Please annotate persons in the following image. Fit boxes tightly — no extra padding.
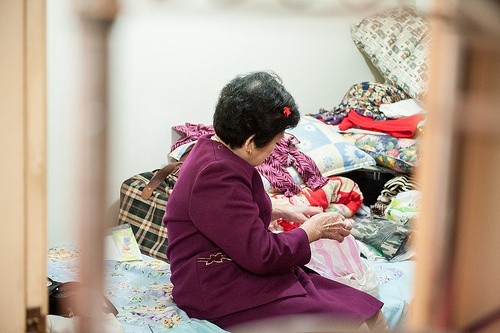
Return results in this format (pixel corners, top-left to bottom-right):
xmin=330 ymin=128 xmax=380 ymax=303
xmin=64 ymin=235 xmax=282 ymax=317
xmin=162 ymin=71 xmax=391 ymax=332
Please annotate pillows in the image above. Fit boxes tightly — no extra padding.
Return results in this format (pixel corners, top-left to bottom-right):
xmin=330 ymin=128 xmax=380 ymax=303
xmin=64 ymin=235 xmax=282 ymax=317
xmin=351 ymin=6 xmax=428 ymax=108
xmin=170 ymin=115 xmax=376 ymax=198
xmin=356 ymin=134 xmax=417 ymax=175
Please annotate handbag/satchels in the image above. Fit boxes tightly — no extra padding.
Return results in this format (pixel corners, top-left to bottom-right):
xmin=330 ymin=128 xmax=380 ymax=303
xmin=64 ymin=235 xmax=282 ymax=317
xmin=118 ymin=160 xmax=186 ymax=262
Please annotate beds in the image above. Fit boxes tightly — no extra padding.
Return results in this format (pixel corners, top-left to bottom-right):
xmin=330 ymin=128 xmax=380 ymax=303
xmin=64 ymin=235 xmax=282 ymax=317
xmin=45 ymin=6 xmax=428 ymax=333
